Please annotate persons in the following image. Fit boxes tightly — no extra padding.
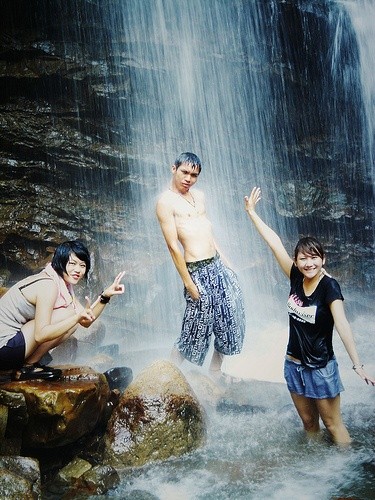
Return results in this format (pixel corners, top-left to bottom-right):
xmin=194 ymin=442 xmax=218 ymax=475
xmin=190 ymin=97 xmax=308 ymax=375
xmin=155 ymin=152 xmax=244 ymax=379
xmin=244 ymin=185 xmax=374 ymax=445
xmin=0 ymin=240 xmax=128 ymax=385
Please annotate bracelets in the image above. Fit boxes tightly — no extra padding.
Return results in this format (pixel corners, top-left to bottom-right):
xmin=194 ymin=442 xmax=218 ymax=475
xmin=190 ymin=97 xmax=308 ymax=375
xmin=96 ymin=291 xmax=110 ymax=305
xmin=350 ymin=362 xmax=364 ymax=369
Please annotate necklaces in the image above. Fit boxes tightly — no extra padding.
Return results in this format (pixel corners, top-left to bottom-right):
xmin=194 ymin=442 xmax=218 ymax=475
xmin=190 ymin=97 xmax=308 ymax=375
xmin=170 ymin=184 xmax=196 ymax=208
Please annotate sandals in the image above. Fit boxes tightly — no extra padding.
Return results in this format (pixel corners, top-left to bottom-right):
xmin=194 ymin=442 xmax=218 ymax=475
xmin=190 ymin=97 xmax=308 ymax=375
xmin=8 ymin=363 xmax=63 ymax=381
